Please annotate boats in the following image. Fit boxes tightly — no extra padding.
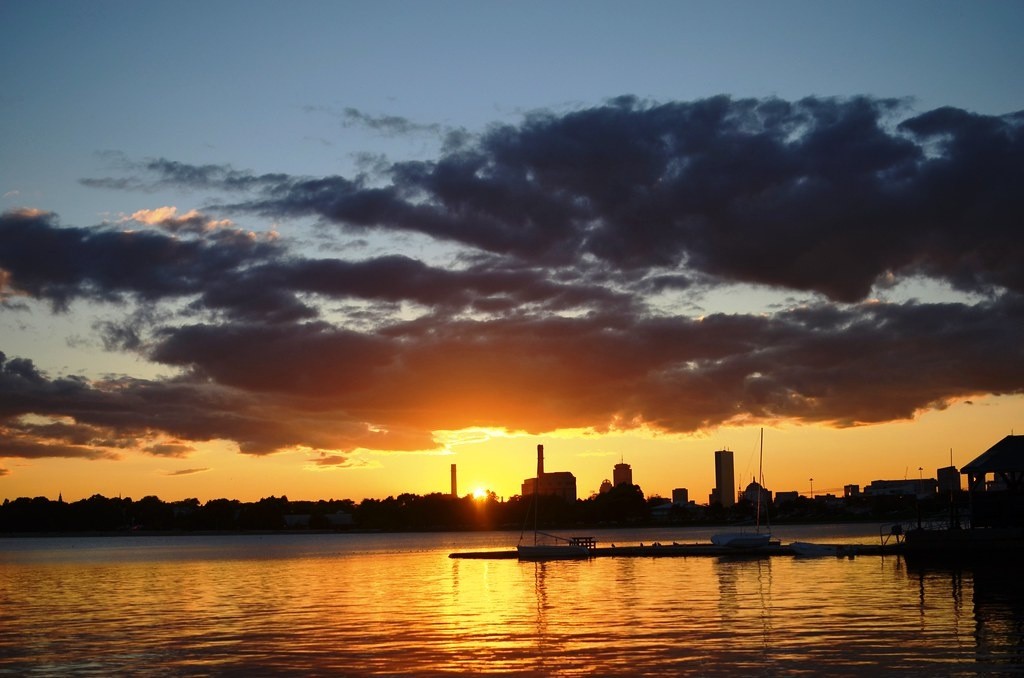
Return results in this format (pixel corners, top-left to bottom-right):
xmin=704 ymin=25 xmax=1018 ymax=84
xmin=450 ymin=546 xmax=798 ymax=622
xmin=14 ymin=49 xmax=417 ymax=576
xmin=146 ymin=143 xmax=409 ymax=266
xmin=904 ymin=525 xmax=1024 ymax=571
xmin=787 ymin=542 xmax=862 ymax=559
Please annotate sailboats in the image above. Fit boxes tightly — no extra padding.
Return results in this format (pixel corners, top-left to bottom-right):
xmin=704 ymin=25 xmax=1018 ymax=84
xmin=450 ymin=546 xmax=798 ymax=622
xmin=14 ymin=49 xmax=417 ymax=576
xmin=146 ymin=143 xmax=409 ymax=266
xmin=517 ymin=478 xmax=591 ymax=560
xmin=710 ymin=429 xmax=776 ymax=549
